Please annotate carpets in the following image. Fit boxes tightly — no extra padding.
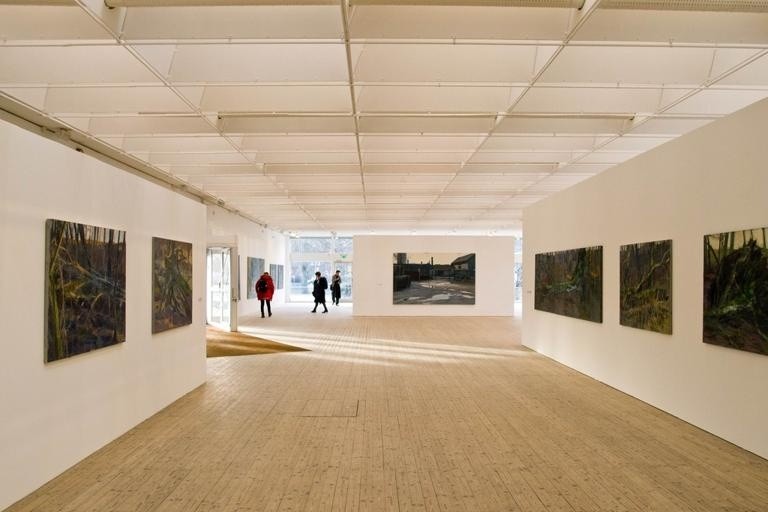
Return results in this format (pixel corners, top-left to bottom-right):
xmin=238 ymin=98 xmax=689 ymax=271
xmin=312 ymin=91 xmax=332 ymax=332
xmin=204 ymin=324 xmax=314 ymax=359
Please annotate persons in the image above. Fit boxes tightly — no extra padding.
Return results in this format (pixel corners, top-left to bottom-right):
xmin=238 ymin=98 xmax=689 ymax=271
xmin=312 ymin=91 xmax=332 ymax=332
xmin=332 ymin=270 xmax=341 ymax=305
xmin=311 ymin=271 xmax=328 ymax=313
xmin=256 ymin=272 xmax=274 ymax=318
